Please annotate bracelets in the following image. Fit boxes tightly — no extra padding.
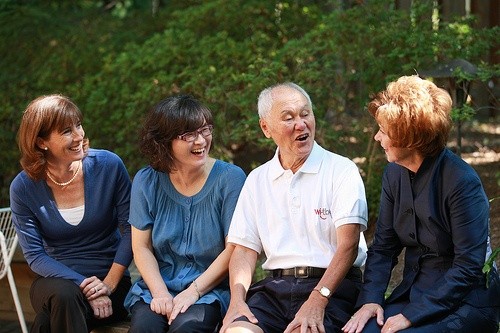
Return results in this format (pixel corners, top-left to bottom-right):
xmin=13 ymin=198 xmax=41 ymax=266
xmin=189 ymin=280 xmax=204 ymax=300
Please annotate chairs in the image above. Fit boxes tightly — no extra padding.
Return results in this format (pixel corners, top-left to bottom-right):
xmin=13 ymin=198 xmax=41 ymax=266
xmin=0 ymin=208 xmax=30 ymax=333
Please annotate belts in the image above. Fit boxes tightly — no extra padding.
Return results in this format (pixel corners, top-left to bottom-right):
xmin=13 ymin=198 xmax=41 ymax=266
xmin=269 ymin=267 xmax=350 ymax=279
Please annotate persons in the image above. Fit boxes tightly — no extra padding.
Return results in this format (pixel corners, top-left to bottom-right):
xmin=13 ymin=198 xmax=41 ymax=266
xmin=127 ymin=96 xmax=247 ymax=333
xmin=340 ymin=74 xmax=499 ymax=333
xmin=11 ymin=93 xmax=135 ymax=333
xmin=218 ymin=80 xmax=368 ymax=332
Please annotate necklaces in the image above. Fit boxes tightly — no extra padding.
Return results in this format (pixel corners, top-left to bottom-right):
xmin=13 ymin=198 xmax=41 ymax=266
xmin=45 ymin=160 xmax=83 ymax=187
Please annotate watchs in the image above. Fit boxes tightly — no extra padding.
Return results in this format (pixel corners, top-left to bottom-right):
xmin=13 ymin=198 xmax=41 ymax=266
xmin=314 ymin=284 xmax=333 ymax=300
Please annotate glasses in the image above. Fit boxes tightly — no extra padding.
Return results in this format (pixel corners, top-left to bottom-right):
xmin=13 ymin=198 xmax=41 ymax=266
xmin=176 ymin=123 xmax=213 ymax=142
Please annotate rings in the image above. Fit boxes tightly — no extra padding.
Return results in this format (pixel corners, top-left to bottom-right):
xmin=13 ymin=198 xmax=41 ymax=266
xmin=93 ymin=286 xmax=98 ymax=293
xmin=388 ymin=326 xmax=393 ymax=331
xmin=350 ymin=315 xmax=356 ymax=320
